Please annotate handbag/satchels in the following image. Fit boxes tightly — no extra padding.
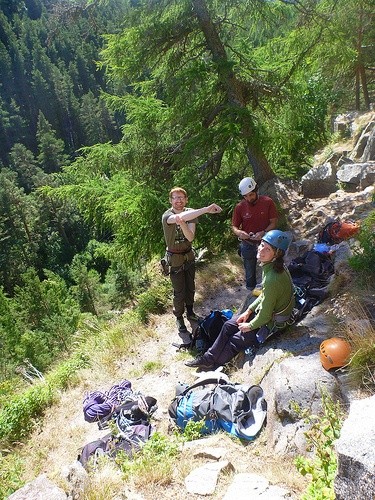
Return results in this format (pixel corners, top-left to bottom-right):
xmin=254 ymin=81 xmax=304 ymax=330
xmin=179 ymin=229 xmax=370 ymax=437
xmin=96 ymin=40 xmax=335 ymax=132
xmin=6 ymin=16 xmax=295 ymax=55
xmin=79 ymin=378 xmax=159 ymax=472
xmin=317 ymin=221 xmax=359 ymax=245
xmin=192 ymin=310 xmax=233 ymax=353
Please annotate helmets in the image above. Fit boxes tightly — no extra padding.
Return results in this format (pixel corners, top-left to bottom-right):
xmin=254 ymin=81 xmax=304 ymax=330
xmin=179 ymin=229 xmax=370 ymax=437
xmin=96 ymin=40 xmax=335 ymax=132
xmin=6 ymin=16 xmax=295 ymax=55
xmin=239 ymin=177 xmax=257 ymax=195
xmin=262 ymin=230 xmax=290 ymax=252
xmin=320 ymin=338 xmax=351 ymax=371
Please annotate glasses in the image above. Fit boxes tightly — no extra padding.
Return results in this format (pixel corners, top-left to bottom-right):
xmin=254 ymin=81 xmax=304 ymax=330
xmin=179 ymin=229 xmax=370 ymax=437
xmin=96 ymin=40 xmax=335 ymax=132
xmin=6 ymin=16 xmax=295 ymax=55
xmin=171 ymin=196 xmax=186 ymax=200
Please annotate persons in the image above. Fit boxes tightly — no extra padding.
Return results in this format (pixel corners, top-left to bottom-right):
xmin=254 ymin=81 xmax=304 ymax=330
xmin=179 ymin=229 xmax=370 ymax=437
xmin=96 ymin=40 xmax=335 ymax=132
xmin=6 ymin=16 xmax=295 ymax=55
xmin=162 ymin=188 xmax=223 ymax=331
xmin=183 ymin=229 xmax=296 ymax=372
xmin=231 ymin=177 xmax=278 ymax=293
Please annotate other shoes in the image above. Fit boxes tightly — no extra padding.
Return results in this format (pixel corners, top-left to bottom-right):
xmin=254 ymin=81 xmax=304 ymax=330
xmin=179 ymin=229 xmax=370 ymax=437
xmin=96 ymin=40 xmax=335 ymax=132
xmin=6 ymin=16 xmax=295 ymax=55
xmin=176 ymin=318 xmax=187 ymax=332
xmin=186 ymin=312 xmax=203 ymax=321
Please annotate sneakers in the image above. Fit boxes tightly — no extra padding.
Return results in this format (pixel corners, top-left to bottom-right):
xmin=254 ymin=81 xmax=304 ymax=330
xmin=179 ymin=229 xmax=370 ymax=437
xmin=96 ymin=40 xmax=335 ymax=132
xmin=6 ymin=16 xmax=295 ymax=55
xmin=184 ymin=354 xmax=213 ymax=368
xmin=200 ymin=364 xmax=223 ymax=370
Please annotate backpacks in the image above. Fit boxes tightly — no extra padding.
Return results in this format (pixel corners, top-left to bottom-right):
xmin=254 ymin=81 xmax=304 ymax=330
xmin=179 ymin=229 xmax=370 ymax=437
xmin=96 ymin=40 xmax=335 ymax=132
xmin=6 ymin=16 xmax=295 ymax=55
xmin=168 ymin=372 xmax=268 ymax=441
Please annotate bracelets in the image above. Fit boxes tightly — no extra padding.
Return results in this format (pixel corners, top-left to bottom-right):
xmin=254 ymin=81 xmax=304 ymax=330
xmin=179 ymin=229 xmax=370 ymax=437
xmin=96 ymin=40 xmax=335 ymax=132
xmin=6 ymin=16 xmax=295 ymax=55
xmin=248 ymin=323 xmax=252 ymax=331
xmin=264 ymin=230 xmax=266 ymax=232
